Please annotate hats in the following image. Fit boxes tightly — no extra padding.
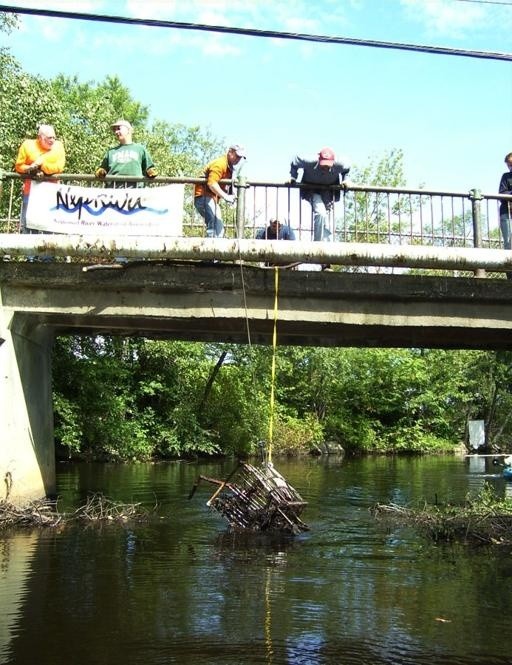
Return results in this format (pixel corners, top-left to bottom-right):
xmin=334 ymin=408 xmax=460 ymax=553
xmin=109 ymin=119 xmax=133 ymax=128
xmin=319 ymin=146 xmax=335 ymax=166
xmin=229 ymin=142 xmax=248 ymax=160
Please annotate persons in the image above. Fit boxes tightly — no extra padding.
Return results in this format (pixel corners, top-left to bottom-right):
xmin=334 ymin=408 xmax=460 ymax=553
xmin=285 ymin=150 xmax=350 ymax=240
xmin=15 ymin=125 xmax=66 ymax=234
xmin=256 ymin=216 xmax=295 ymax=240
xmin=94 ymin=119 xmax=158 ymax=189
xmin=194 ymin=145 xmax=247 ymax=238
xmin=498 ymin=152 xmax=512 ymax=250
